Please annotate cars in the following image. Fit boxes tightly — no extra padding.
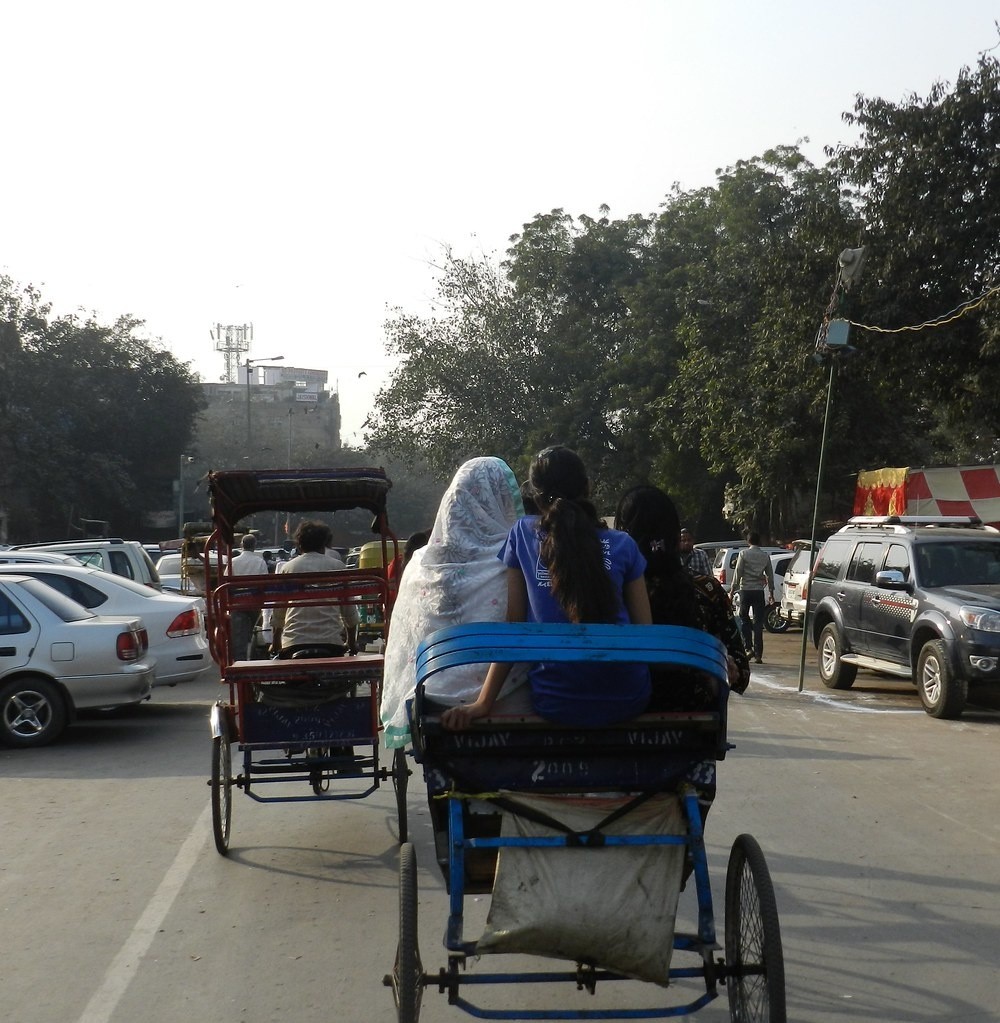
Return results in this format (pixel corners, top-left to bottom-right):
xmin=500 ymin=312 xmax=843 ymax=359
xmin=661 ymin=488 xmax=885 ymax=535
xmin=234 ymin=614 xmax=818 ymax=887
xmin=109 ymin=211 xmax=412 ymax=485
xmin=1 ymin=537 xmax=230 ymax=632
xmin=692 ymin=537 xmax=826 ymax=632
xmin=0 ymin=563 xmax=214 ymax=693
xmin=0 ymin=573 xmax=161 ymax=748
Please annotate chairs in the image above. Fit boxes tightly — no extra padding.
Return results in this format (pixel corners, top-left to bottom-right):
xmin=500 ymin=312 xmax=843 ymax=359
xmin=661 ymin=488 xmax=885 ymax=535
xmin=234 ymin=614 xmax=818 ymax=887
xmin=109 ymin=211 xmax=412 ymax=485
xmin=929 ymin=548 xmax=958 ymax=579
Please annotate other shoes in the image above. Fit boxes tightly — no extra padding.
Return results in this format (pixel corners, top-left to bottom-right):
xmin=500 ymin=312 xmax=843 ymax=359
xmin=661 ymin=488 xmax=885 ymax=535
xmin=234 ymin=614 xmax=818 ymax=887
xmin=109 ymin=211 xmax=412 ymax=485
xmin=755 ymin=657 xmax=762 ymax=663
xmin=283 ymin=746 xmax=304 ymax=755
xmin=339 ymin=764 xmax=363 ymax=778
xmin=746 ymin=649 xmax=754 ymax=660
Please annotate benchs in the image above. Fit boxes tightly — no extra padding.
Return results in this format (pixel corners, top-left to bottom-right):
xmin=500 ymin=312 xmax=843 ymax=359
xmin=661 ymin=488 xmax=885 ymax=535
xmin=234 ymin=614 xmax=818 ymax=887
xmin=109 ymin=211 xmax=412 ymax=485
xmin=417 ymin=703 xmax=723 ymax=826
xmin=226 ymin=654 xmax=385 ymax=744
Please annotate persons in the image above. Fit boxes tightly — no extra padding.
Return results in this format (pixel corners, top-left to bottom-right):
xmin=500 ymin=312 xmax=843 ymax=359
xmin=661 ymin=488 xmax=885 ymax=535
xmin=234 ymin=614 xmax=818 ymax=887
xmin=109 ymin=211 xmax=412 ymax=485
xmin=263 ymin=549 xmax=286 ymax=572
xmin=325 ymin=534 xmax=341 ymax=560
xmin=439 ymin=447 xmax=653 ymax=732
xmin=268 ymin=520 xmax=363 ymax=775
xmin=223 ymin=534 xmax=268 ymax=659
xmin=380 ymin=457 xmax=527 ymax=751
xmin=614 ymin=485 xmax=750 ymax=710
xmin=730 ymin=535 xmax=775 ymax=663
xmin=679 ymin=529 xmax=714 ymax=578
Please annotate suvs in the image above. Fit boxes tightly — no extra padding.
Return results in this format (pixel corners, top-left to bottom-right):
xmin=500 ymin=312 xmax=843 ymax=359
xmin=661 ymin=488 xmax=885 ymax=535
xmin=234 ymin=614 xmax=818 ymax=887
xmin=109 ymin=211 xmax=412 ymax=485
xmin=803 ymin=515 xmax=1000 ymax=719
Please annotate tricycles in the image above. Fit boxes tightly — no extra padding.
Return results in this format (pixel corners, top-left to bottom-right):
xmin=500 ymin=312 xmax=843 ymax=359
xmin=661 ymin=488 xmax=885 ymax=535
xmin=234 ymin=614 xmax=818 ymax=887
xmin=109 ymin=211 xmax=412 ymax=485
xmin=381 ymin=623 xmax=787 ymax=1022
xmin=182 ymin=462 xmax=415 ymax=854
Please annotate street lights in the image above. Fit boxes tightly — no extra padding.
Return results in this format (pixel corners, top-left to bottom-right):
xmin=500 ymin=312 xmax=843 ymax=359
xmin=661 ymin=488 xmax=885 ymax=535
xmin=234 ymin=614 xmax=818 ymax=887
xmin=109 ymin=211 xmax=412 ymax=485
xmin=246 ymin=356 xmax=286 ymax=460
